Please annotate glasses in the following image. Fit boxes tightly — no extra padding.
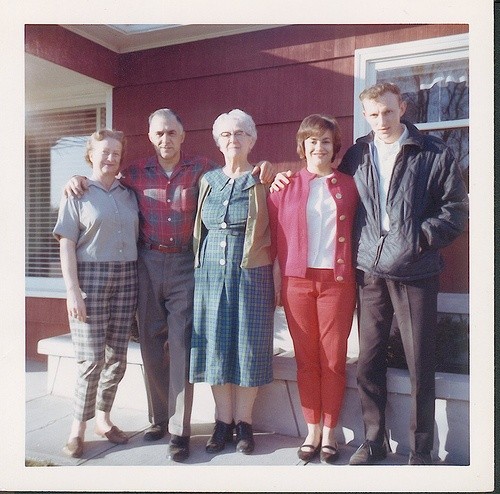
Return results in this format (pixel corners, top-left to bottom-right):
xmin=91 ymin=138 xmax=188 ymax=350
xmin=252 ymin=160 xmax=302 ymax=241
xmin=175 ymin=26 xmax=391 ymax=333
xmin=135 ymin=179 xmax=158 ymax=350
xmin=221 ymin=131 xmax=252 ymax=138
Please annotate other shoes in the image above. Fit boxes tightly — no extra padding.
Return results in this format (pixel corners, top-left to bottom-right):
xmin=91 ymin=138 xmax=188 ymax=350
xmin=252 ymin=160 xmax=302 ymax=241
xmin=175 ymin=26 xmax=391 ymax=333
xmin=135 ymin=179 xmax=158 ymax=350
xmin=144 ymin=424 xmax=167 ymax=440
xmin=320 ymin=441 xmax=338 ymax=461
xmin=409 ymin=450 xmax=432 ymax=465
xmin=94 ymin=425 xmax=128 ymax=444
xmin=63 ymin=437 xmax=85 ymax=459
xmin=349 ymin=439 xmax=387 ymax=465
xmin=169 ymin=434 xmax=190 ymax=462
xmin=206 ymin=417 xmax=235 ymax=451
xmin=235 ymin=419 xmax=254 ymax=453
xmin=297 ymin=432 xmax=321 ymax=459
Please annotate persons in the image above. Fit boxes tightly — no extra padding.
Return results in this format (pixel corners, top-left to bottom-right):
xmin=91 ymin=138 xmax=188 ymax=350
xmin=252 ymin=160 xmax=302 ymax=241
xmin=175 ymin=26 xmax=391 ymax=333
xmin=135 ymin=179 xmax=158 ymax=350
xmin=51 ymin=129 xmax=131 ymax=459
xmin=263 ymin=111 xmax=367 ymax=463
xmin=182 ymin=112 xmax=281 ymax=455
xmin=266 ymin=84 xmax=469 ymax=465
xmin=65 ymin=108 xmax=278 ymax=463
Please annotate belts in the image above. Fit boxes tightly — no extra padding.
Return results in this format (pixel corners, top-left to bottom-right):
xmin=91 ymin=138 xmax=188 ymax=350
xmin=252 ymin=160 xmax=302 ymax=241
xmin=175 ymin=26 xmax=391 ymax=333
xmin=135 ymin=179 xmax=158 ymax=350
xmin=138 ymin=242 xmax=193 ymax=253
